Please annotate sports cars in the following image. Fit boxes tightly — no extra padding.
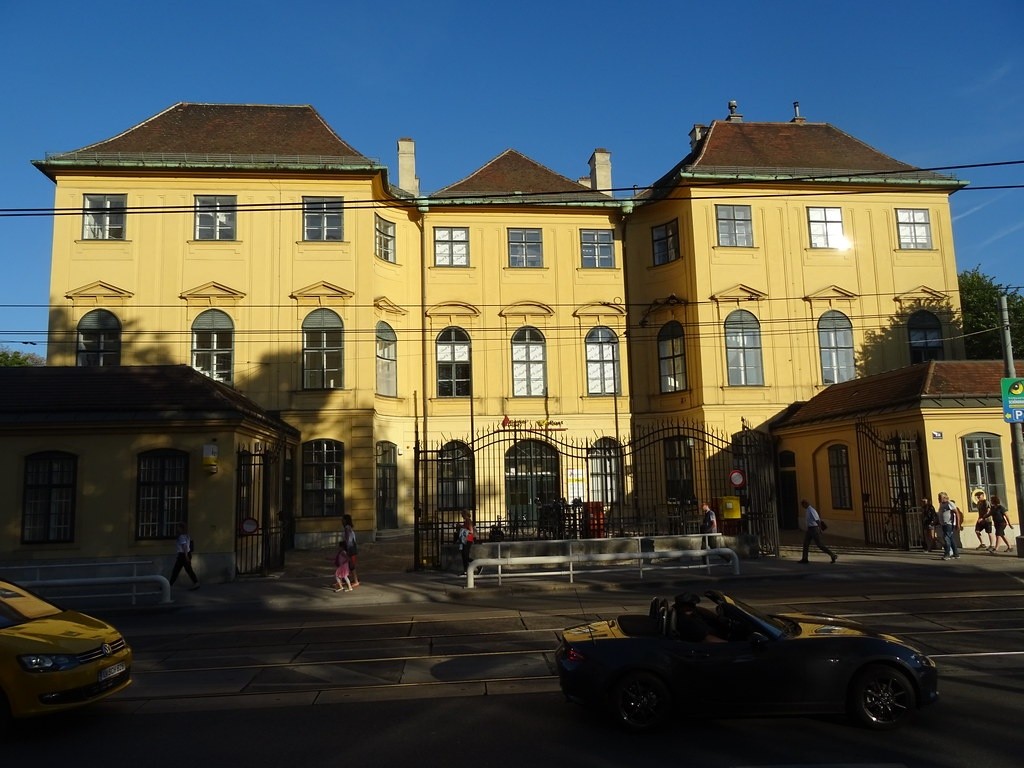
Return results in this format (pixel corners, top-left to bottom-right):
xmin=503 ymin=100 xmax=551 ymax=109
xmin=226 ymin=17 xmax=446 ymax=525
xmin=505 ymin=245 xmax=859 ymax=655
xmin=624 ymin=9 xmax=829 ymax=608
xmin=555 ymin=588 xmax=938 ymax=732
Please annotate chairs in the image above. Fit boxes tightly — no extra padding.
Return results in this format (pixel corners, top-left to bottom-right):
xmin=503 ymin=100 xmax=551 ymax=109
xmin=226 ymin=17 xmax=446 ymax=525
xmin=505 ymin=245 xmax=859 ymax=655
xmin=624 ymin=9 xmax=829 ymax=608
xmin=623 ymin=597 xmax=681 ymax=638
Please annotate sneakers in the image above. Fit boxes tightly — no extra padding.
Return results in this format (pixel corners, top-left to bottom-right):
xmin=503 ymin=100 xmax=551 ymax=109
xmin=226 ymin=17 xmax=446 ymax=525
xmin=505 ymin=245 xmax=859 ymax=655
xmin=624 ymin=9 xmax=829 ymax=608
xmin=830 ymin=555 xmax=837 ymax=563
xmin=989 ymin=548 xmax=998 ymax=554
xmin=986 ymin=546 xmax=994 ymax=550
xmin=976 ymin=544 xmax=987 ymax=550
xmin=477 ymin=566 xmax=483 ymax=574
xmin=950 ymin=555 xmax=960 ymax=559
xmin=941 ymin=556 xmax=951 ymax=560
xmin=1003 ymin=548 xmax=1014 ymax=552
xmin=797 ymin=559 xmax=809 ymax=564
xmin=457 ymin=573 xmax=467 ymax=578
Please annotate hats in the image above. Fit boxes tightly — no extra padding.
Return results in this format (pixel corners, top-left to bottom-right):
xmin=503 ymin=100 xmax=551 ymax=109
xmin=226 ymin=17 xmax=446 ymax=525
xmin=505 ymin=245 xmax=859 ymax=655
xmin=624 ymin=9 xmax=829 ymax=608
xmin=674 ymin=592 xmax=701 ymax=607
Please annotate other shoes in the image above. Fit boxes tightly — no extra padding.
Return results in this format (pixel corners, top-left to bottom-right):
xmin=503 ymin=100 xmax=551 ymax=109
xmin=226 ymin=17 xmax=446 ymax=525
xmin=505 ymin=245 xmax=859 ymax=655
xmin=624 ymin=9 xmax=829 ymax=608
xmin=189 ymin=584 xmax=200 ymax=591
xmin=345 ymin=588 xmax=353 ymax=592
xmin=352 ymin=583 xmax=360 ymax=588
xmin=335 ymin=587 xmax=343 ymax=593
xmin=331 ymin=583 xmax=345 ymax=587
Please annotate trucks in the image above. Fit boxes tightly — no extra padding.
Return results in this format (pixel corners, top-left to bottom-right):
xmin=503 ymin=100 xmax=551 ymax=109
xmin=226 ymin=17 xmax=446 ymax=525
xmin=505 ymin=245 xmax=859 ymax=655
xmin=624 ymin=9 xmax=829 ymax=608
xmin=667 ymin=498 xmax=691 ymax=506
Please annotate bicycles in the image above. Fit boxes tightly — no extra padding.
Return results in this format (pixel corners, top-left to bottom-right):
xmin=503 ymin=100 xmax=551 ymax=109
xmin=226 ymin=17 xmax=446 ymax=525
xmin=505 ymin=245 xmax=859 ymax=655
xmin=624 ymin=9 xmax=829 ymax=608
xmin=500 ymin=510 xmax=537 ymax=536
xmin=563 ymin=507 xmax=574 ymax=528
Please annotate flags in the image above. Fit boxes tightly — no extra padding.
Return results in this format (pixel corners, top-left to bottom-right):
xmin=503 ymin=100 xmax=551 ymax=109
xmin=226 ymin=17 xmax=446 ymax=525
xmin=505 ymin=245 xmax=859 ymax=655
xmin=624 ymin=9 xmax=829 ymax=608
xmin=502 ymin=415 xmax=510 ymax=428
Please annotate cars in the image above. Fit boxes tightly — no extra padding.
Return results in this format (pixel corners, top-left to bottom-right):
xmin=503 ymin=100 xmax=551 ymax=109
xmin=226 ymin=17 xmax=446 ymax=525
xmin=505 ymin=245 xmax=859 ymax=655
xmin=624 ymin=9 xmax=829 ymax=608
xmin=0 ymin=583 xmax=132 ymax=729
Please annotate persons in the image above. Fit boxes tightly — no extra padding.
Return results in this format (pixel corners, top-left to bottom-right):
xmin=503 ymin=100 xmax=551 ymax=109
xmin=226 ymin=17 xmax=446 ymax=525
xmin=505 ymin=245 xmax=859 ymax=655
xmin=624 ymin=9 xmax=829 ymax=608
xmin=938 ymin=491 xmax=960 ymax=561
xmin=532 ymin=498 xmax=568 ymax=539
xmin=169 ymin=522 xmax=200 ymax=590
xmin=701 ymin=501 xmax=733 ymax=572
xmin=675 ymin=592 xmax=727 ymax=644
xmin=333 ymin=515 xmax=359 ymax=592
xmin=975 ymin=492 xmax=1014 ymax=554
xmin=797 ymin=499 xmax=839 ymax=564
xmin=459 ymin=510 xmax=484 ymax=577
xmin=921 ymin=496 xmax=935 ymax=553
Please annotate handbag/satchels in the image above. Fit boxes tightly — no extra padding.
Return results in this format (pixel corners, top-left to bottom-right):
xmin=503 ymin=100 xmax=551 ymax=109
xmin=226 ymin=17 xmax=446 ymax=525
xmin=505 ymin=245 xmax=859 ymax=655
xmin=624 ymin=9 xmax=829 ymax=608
xmin=347 ymin=544 xmax=358 ymax=556
xmin=190 ymin=539 xmax=194 ymax=552
xmin=821 ymin=521 xmax=827 ymax=531
